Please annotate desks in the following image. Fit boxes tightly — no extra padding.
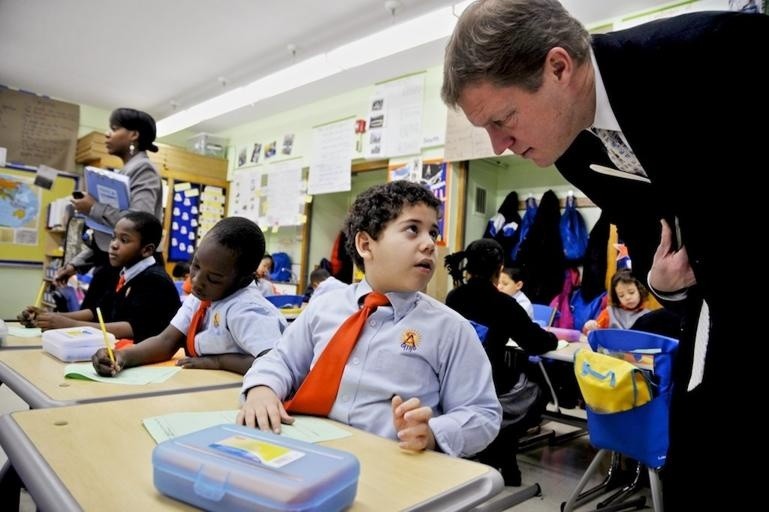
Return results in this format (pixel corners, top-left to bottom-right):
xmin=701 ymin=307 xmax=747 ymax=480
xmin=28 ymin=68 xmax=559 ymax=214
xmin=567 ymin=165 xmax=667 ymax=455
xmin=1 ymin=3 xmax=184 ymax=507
xmin=1 ymin=306 xmax=579 ymax=510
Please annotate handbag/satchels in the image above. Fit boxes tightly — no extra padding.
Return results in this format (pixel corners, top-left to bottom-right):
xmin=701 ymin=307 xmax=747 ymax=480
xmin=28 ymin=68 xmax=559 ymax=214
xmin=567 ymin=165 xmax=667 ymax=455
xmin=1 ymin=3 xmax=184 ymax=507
xmin=573 ymin=349 xmax=659 ymax=417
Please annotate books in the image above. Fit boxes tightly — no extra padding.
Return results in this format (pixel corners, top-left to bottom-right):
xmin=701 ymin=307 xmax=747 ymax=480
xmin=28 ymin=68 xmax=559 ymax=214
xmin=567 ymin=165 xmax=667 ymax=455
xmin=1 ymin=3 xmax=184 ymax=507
xmin=84 ymin=166 xmax=130 ymax=235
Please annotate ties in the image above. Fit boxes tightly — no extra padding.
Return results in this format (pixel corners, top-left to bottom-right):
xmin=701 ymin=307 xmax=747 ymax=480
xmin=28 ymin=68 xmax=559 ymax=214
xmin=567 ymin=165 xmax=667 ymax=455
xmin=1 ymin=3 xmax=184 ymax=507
xmin=283 ymin=290 xmax=390 ymax=418
xmin=185 ymin=299 xmax=213 ymax=359
xmin=592 ymin=125 xmax=648 ymax=179
xmin=115 ymin=272 xmax=125 ymax=293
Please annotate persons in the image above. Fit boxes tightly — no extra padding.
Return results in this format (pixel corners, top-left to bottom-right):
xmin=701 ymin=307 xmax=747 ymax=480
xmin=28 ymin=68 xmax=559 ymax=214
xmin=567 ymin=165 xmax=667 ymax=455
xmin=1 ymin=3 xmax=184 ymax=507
xmin=444 ymin=239 xmax=558 ymax=438
xmin=496 ymin=267 xmax=534 ymax=322
xmin=19 ymin=212 xmax=182 ymax=344
xmin=248 ymin=252 xmax=274 ymax=296
xmin=173 ymin=262 xmax=192 ymax=296
xmin=236 ymin=180 xmax=503 ymax=459
xmin=52 ymin=108 xmax=164 ymax=310
xmin=439 ymin=0 xmax=767 ymax=512
xmin=92 ymin=216 xmax=289 ymax=377
xmin=308 ymin=267 xmax=349 ymax=304
xmin=583 ymin=268 xmax=652 ymax=333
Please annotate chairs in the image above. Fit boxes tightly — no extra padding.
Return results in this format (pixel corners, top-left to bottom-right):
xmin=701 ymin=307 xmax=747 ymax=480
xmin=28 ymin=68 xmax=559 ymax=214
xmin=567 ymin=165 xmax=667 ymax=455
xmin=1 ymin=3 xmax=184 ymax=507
xmin=561 ymin=328 xmax=684 ymax=512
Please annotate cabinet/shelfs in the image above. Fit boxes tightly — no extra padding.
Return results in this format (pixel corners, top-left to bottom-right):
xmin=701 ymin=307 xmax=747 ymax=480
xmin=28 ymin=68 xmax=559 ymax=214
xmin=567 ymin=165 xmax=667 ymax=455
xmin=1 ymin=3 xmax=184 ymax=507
xmin=84 ymin=158 xmax=229 ymax=278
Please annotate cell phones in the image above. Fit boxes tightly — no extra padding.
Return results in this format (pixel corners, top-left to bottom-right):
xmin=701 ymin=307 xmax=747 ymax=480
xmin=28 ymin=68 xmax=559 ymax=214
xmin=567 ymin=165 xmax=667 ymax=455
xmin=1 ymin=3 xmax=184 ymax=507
xmin=72 ymin=191 xmax=84 ymax=199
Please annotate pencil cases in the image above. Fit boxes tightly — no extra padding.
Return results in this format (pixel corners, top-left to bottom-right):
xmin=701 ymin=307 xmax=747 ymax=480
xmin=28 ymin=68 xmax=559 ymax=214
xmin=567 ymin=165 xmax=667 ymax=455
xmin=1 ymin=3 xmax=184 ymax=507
xmin=153 ymin=423 xmax=360 ymax=512
xmin=42 ymin=326 xmax=116 ymax=362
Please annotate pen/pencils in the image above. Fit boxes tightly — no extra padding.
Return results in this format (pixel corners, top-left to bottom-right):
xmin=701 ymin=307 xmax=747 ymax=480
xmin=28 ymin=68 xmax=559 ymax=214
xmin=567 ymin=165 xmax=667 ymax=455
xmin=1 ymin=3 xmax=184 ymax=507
xmin=96 ymin=307 xmax=116 ymax=370
xmin=27 ymin=281 xmax=46 ymax=320
xmin=546 ymin=302 xmax=559 ymax=331
